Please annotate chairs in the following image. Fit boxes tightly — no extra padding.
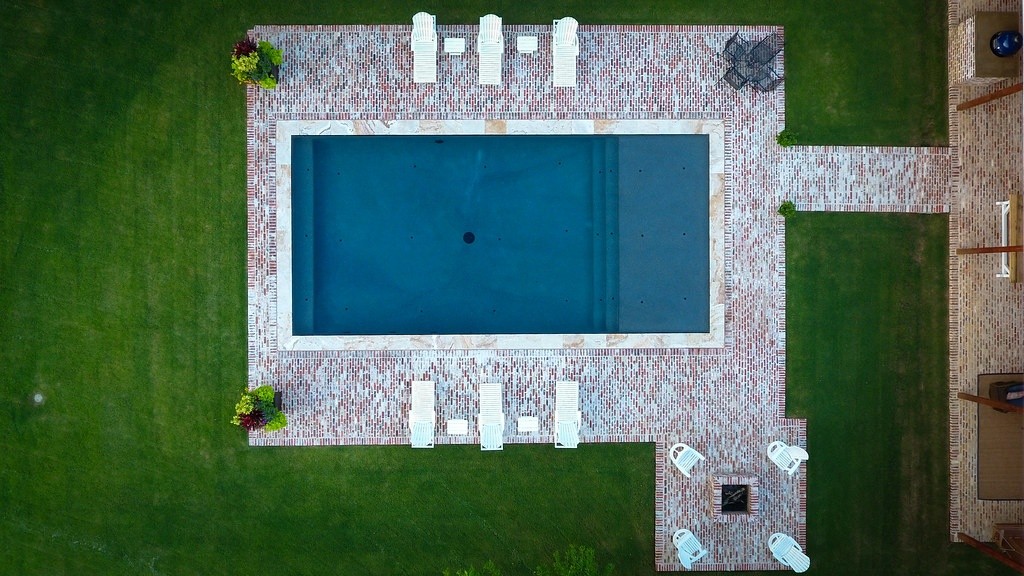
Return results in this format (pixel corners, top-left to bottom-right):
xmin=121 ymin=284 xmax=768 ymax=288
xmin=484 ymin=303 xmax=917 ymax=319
xmin=768 ymin=532 xmax=811 ymax=573
xmin=673 ymin=529 xmax=709 ymax=570
xmin=670 ymin=443 xmax=706 ymax=478
xmin=477 ymin=14 xmax=505 ymax=84
xmin=713 ymin=66 xmax=750 ymax=100
xmin=408 ymin=380 xmax=436 ymax=449
xmin=553 ymin=17 xmax=579 ymax=88
xmin=767 ymin=440 xmax=810 ymax=475
xmin=723 ymin=29 xmax=749 ymax=64
xmin=750 ymin=32 xmax=785 ymax=65
xmin=476 ymin=382 xmax=505 ymax=450
xmin=410 ymin=11 xmax=437 ymax=83
xmin=553 ymin=380 xmax=582 ymax=449
xmin=744 ymin=63 xmax=784 ymax=94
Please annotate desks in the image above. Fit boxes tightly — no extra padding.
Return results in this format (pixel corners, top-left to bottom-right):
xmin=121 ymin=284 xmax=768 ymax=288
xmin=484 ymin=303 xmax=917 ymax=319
xmin=447 ymin=419 xmax=468 ymax=435
xmin=444 ymin=38 xmax=465 ymax=61
xmin=517 ymin=36 xmax=539 ymax=58
xmin=733 ymin=40 xmax=775 ymax=82
xmin=517 ymin=415 xmax=539 ymax=432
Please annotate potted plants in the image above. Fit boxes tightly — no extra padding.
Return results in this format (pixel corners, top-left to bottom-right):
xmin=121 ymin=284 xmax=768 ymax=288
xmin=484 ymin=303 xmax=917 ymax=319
xmin=230 ymin=384 xmax=288 ymax=433
xmin=777 ymin=128 xmax=799 ymax=149
xmin=227 ymin=35 xmax=284 ymax=90
xmin=776 ymin=199 xmax=796 ymax=219
